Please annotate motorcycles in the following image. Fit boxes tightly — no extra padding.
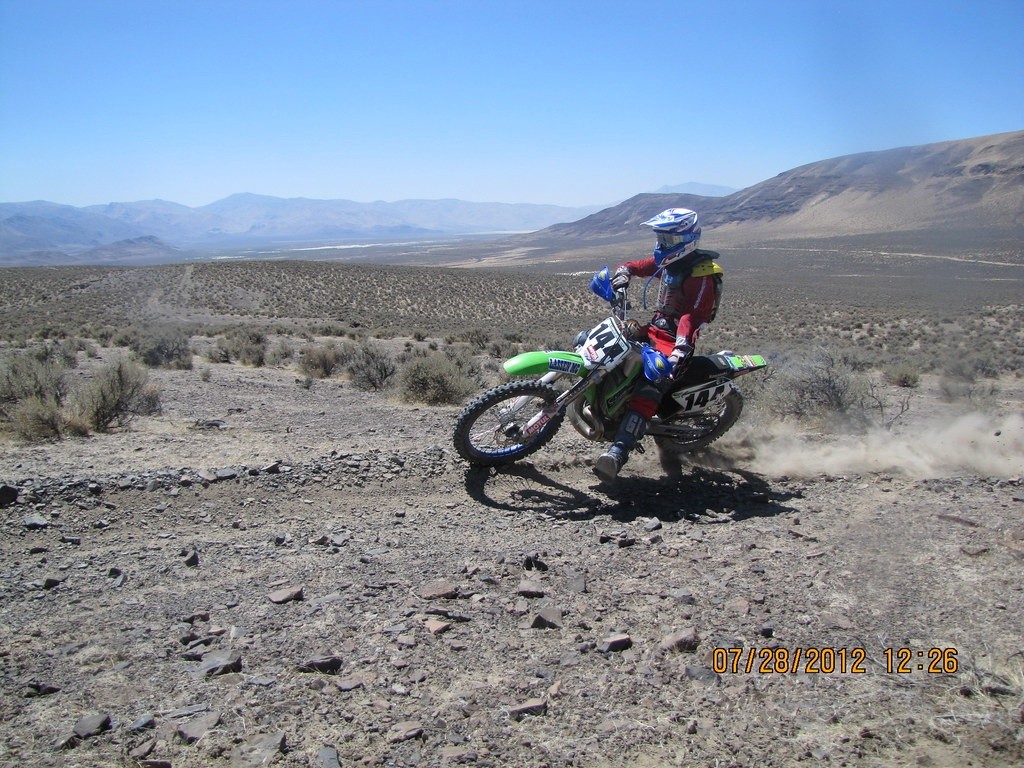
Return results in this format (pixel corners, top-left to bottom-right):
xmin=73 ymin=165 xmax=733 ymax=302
xmin=450 ymin=273 xmax=767 ymax=470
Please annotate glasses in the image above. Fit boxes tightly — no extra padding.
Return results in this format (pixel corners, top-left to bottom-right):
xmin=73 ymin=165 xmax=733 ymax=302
xmin=655 ymin=228 xmax=702 ymax=248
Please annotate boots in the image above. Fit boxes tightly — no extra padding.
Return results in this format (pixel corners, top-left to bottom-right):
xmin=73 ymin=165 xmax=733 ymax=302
xmin=596 ymin=411 xmax=647 ymax=487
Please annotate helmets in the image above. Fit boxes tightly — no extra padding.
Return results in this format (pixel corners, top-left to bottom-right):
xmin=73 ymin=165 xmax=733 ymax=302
xmin=639 ymin=208 xmax=701 ymax=267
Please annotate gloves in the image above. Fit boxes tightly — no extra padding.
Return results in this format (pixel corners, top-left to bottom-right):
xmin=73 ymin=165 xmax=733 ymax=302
xmin=611 ymin=266 xmax=631 ymax=293
xmin=667 ymin=346 xmax=694 ymax=382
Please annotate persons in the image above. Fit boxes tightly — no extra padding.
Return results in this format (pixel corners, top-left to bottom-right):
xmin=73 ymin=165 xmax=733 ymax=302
xmin=594 ymin=207 xmax=725 ymax=485
